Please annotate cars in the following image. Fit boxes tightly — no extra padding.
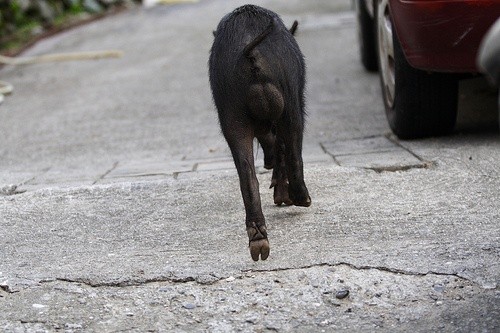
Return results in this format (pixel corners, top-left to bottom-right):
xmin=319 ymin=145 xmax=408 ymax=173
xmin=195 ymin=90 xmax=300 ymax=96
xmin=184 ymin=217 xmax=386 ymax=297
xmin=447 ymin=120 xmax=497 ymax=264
xmin=351 ymin=2 xmax=499 ymax=140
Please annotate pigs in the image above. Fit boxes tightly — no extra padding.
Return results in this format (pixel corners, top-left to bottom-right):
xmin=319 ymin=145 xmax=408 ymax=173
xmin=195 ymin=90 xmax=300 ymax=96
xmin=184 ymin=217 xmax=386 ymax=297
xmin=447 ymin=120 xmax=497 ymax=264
xmin=207 ymin=4 xmax=312 ymax=261
